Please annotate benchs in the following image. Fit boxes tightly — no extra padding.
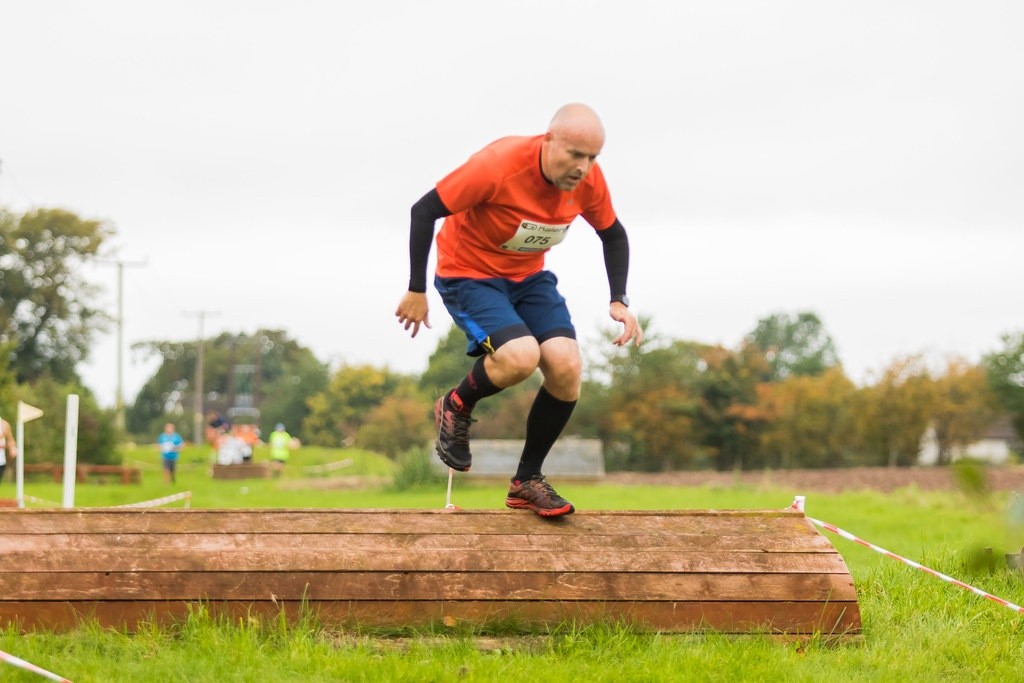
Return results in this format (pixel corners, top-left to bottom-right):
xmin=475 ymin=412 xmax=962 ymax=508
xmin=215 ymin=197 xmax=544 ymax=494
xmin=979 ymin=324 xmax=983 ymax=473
xmin=213 ymin=464 xmax=264 ymax=479
xmin=13 ymin=462 xmax=139 ymax=483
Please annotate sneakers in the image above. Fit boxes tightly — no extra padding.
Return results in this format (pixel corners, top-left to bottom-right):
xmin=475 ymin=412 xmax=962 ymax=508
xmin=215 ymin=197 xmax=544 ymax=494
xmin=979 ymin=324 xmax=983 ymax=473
xmin=506 ymin=472 xmax=575 ymax=517
xmin=434 ymin=388 xmax=479 ymax=472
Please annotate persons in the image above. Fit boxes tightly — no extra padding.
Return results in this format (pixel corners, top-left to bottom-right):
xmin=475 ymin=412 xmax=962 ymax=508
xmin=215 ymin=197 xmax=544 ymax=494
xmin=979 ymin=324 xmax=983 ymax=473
xmin=395 ymin=103 xmax=644 ymax=516
xmin=206 ymin=410 xmax=257 ymax=466
xmin=267 ymin=423 xmax=292 ymax=477
xmin=157 ymin=423 xmax=184 ymax=485
xmin=0 ymin=418 xmax=16 ymax=482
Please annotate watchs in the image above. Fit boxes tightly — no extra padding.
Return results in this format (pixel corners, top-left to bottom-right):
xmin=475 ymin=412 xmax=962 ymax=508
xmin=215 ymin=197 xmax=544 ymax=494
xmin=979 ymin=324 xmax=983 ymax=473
xmin=611 ymin=295 xmax=629 ymax=307
